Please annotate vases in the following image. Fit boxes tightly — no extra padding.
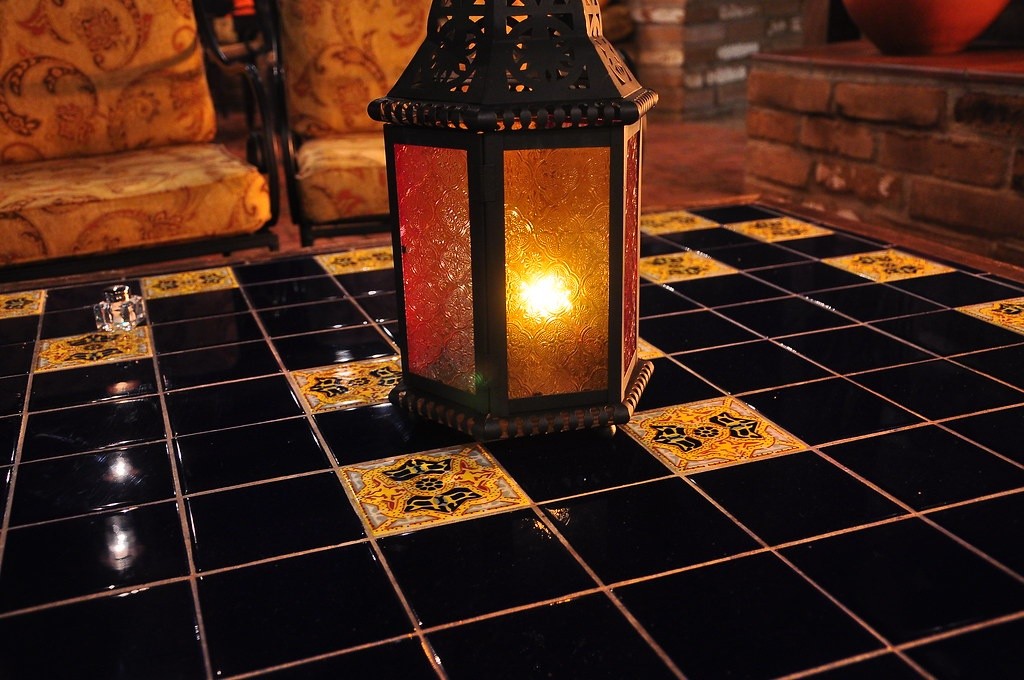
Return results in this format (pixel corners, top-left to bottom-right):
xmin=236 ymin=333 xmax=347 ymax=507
xmin=843 ymin=0 xmax=1012 ymax=58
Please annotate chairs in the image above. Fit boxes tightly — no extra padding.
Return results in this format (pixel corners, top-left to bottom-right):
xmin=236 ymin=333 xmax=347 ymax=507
xmin=0 ymin=0 xmax=432 ymax=284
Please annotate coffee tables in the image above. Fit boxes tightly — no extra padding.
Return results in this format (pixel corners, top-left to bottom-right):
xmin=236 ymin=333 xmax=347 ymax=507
xmin=0 ymin=191 xmax=1023 ymax=680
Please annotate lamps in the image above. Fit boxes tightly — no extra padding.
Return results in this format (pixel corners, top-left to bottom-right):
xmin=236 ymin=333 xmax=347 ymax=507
xmin=368 ymin=0 xmax=658 ymax=443
